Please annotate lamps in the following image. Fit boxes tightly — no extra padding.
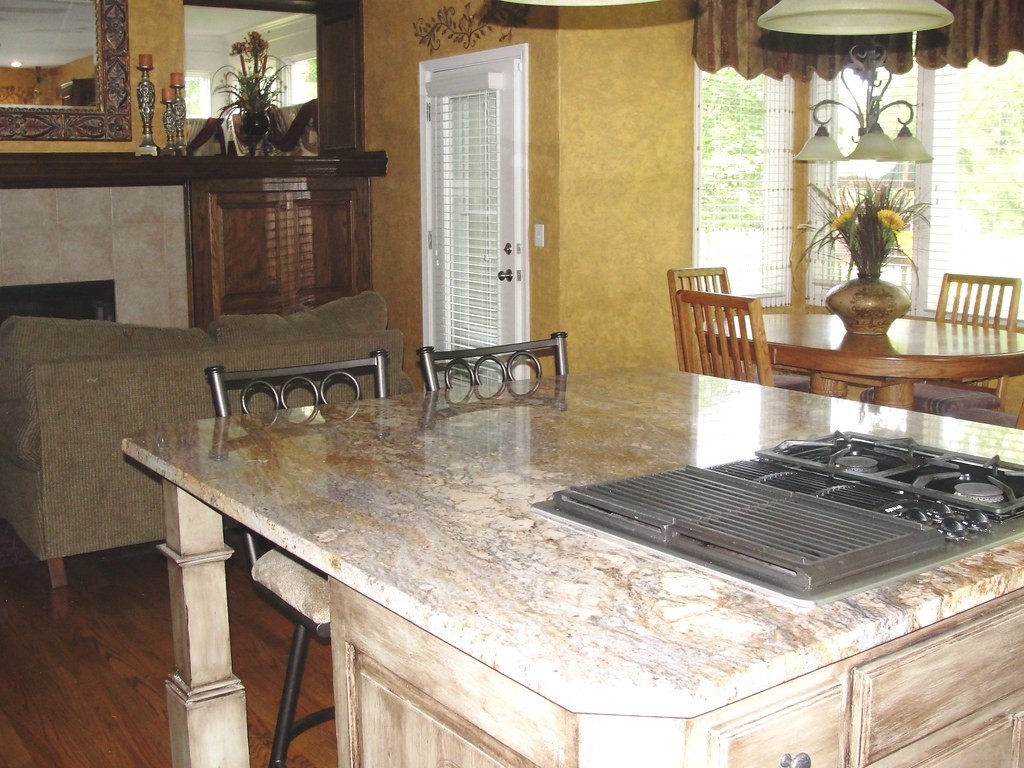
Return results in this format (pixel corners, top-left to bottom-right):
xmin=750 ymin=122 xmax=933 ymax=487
xmin=757 ymin=0 xmax=954 ymax=36
xmin=793 ymin=42 xmax=933 ymax=164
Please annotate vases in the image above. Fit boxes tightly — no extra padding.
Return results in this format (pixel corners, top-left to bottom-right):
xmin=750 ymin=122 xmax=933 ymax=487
xmin=243 ymin=113 xmax=270 ymax=157
xmin=824 ymin=271 xmax=912 ymax=335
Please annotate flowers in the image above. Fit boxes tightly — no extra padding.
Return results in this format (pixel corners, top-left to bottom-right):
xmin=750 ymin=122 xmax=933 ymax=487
xmin=810 ymin=176 xmax=928 ymax=274
xmin=217 ymin=32 xmax=283 ymax=109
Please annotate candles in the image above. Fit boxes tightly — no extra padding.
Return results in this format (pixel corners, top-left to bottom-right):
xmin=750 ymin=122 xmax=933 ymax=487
xmin=138 ymin=54 xmax=152 ymax=67
xmin=170 ymin=72 xmax=184 ymax=85
xmin=161 ymin=87 xmax=176 ymax=102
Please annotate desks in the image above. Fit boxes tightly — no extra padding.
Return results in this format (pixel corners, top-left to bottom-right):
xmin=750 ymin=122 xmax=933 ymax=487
xmin=699 ymin=313 xmax=1024 ymax=407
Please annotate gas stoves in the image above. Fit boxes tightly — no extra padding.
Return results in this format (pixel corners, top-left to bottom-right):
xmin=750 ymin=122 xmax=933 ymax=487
xmin=530 ymin=429 xmax=1024 ymax=607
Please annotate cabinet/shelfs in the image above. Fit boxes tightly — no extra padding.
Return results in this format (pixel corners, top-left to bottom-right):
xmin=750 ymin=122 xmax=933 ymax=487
xmin=326 ymin=574 xmax=581 ymax=768
xmin=684 ymin=593 xmax=1024 ymax=768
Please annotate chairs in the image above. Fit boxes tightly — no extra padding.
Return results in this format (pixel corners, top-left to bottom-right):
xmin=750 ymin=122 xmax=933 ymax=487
xmin=415 ymin=330 xmax=567 ymax=391
xmin=676 ymin=290 xmax=811 ymax=392
xmin=858 ymin=272 xmax=1022 ymax=417
xmin=667 ymin=266 xmax=731 ymax=371
xmin=203 ymin=350 xmax=391 ymax=768
xmin=942 ymin=398 xmax=1024 ymax=429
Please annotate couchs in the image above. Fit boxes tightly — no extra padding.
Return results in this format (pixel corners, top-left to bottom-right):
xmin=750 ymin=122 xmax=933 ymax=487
xmin=0 ymin=290 xmax=415 ymax=589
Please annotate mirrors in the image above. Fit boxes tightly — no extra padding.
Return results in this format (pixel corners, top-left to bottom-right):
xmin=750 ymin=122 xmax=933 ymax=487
xmin=0 ymin=0 xmax=133 ymax=142
